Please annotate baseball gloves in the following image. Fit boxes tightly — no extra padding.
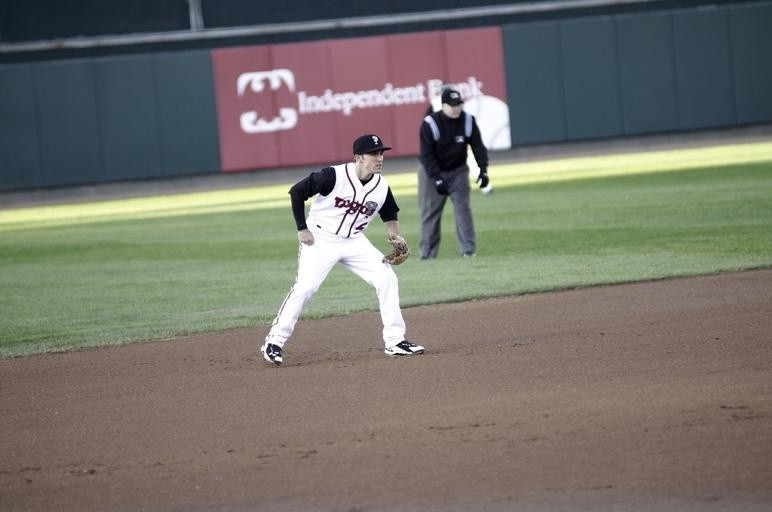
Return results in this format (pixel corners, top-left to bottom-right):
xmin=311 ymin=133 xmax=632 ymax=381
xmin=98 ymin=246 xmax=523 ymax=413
xmin=382 ymin=235 xmax=412 ymax=265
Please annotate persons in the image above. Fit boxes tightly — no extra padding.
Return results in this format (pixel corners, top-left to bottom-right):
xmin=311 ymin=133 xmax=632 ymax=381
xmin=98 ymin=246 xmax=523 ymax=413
xmin=257 ymin=129 xmax=427 ymax=366
xmin=418 ymin=86 xmax=490 ymax=261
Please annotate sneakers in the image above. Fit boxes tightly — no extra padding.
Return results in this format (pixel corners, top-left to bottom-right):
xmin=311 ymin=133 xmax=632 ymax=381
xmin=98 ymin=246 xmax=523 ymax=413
xmin=260 ymin=341 xmax=284 ymax=367
xmin=384 ymin=341 xmax=425 ymax=358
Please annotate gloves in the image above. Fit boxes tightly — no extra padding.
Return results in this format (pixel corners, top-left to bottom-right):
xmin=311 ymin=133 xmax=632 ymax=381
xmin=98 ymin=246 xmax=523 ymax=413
xmin=433 ymin=177 xmax=451 ymax=196
xmin=476 ymin=170 xmax=490 ymax=188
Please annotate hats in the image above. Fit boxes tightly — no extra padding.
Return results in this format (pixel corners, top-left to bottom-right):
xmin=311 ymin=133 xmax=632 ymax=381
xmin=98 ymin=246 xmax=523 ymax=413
xmin=441 ymin=88 xmax=464 ymax=105
xmin=352 ymin=133 xmax=392 ymax=155
